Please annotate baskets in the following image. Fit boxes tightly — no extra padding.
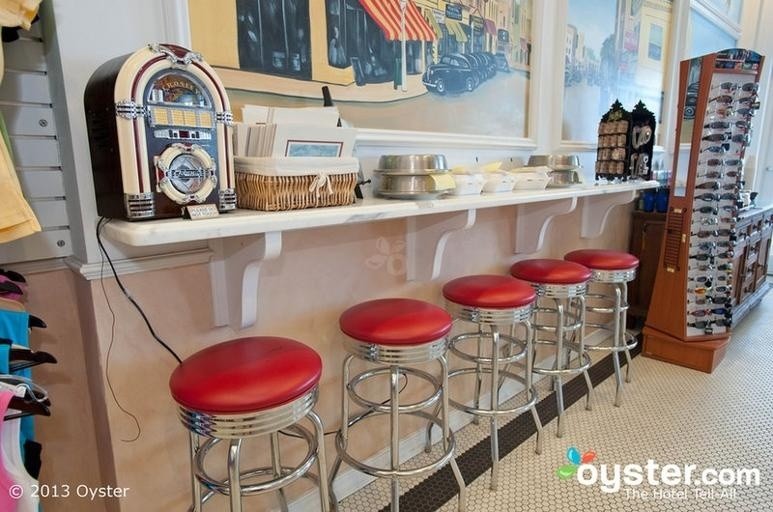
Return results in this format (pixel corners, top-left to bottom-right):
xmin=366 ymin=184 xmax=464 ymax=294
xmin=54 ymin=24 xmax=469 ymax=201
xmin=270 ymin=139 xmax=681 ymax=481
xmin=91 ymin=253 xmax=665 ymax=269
xmin=233 ymin=155 xmax=360 ymax=212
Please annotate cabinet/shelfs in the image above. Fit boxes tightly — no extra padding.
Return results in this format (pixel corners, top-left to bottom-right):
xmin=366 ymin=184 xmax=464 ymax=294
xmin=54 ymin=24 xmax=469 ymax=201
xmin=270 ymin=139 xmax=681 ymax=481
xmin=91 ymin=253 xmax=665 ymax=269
xmin=628 ymin=203 xmax=773 ymax=335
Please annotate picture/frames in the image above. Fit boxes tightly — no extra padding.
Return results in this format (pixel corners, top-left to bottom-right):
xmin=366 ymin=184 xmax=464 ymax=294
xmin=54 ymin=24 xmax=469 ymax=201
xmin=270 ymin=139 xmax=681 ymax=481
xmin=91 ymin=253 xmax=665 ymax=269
xmin=162 ymin=0 xmax=546 ymax=153
xmin=670 ymin=0 xmax=761 ymax=154
xmin=678 ymin=58 xmax=702 ymax=149
xmin=699 ymin=0 xmax=747 ymax=32
xmin=556 ymin=0 xmax=682 ymax=154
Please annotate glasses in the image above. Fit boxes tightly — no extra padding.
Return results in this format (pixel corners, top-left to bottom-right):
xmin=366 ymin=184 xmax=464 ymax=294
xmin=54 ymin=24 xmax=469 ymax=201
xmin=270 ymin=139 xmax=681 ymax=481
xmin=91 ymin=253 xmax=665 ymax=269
xmin=707 ymin=158 xmax=723 ymax=166
xmin=720 ymin=206 xmax=736 ymax=211
xmin=694 ymin=207 xmax=718 ymax=215
xmin=697 ymin=171 xmax=721 ymax=178
xmin=703 ymin=146 xmax=724 ymax=155
xmin=725 ymin=159 xmax=742 ymax=166
xmin=688 ymin=308 xmax=712 ymax=317
xmin=718 ymin=229 xmax=732 ymax=236
xmin=716 ymin=274 xmax=734 ymax=282
xmin=712 ymin=262 xmax=733 ymax=271
xmin=687 ymin=287 xmax=713 ymax=294
xmin=732 ymin=108 xmax=755 ymax=116
xmin=689 ymin=253 xmax=715 ymax=264
xmin=716 ymin=240 xmax=735 ymax=247
xmin=714 ymin=284 xmax=733 ymax=293
xmin=715 ymin=250 xmax=734 ymax=258
xmin=687 ymin=319 xmax=712 ymax=329
xmin=723 ymin=180 xmax=745 ymax=190
xmin=732 ymin=134 xmax=750 ymax=143
xmin=720 ymin=192 xmax=740 ymax=200
xmin=694 ymin=240 xmax=717 ymax=251
xmin=726 ymin=171 xmax=741 ymax=177
xmin=732 ymin=121 xmax=751 ymax=129
xmin=696 ymin=182 xmax=720 ymax=190
xmin=742 ymin=83 xmax=758 ymax=94
xmin=706 ymin=108 xmax=733 ymax=119
xmin=712 ymin=296 xmax=731 ymax=304
xmin=710 ymin=319 xmax=729 ymax=327
xmin=721 ymin=143 xmax=729 ymax=151
xmin=691 ymin=229 xmax=720 ymax=238
xmin=709 ymin=95 xmax=732 ymax=104
xmin=711 ymin=308 xmax=727 ymax=314
xmin=689 ymin=274 xmax=713 ymax=284
xmin=704 ymin=121 xmax=729 ymax=129
xmin=693 ymin=264 xmax=713 ymax=271
xmin=694 ymin=193 xmax=721 ymax=201
xmin=702 ymin=134 xmax=725 ymax=142
xmin=734 ymin=97 xmax=760 ymax=109
xmin=720 ymin=217 xmax=737 ymax=223
xmin=699 ymin=217 xmax=718 ymax=225
xmin=712 ymin=82 xmax=741 ymax=91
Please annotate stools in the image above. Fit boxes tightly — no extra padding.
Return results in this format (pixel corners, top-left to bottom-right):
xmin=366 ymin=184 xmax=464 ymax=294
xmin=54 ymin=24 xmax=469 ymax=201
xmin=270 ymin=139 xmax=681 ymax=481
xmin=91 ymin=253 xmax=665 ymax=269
xmin=327 ymin=298 xmax=466 ymax=512
xmin=169 ymin=335 xmax=336 ymax=512
xmin=564 ymin=249 xmax=637 ymax=411
xmin=509 ymin=260 xmax=592 ymax=439
xmin=423 ymin=275 xmax=542 ymax=490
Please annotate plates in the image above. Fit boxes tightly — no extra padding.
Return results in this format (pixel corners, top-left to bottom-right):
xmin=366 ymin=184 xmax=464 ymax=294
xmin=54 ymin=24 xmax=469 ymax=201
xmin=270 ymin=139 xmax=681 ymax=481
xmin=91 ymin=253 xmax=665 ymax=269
xmin=374 ymin=187 xmax=453 ymax=199
xmin=546 ymin=182 xmax=583 ymax=188
xmin=524 ymin=164 xmax=584 ymax=171
xmin=372 ymin=168 xmax=454 ymax=176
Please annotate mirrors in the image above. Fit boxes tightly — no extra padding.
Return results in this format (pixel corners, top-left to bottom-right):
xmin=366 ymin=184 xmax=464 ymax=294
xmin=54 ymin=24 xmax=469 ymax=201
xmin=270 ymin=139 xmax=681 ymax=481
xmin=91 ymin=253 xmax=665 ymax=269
xmin=673 ymin=57 xmax=708 ymax=198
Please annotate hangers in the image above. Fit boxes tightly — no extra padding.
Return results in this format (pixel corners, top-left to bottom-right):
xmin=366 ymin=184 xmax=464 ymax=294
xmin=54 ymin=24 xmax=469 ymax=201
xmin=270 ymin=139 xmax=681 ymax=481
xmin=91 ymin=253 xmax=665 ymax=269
xmin=0 ymin=266 xmax=58 ymax=421
